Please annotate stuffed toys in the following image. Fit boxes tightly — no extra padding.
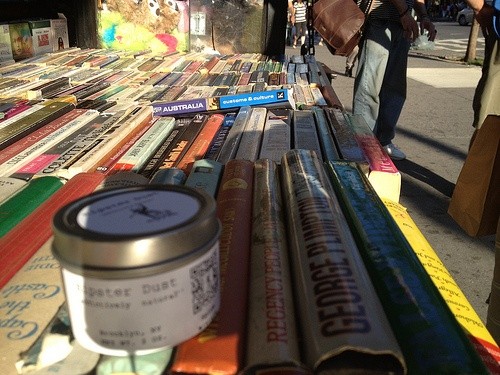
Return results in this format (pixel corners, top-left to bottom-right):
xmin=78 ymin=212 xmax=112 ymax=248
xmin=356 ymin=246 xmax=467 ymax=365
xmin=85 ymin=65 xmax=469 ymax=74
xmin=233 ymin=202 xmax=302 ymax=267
xmin=100 ymin=0 xmax=187 ymax=53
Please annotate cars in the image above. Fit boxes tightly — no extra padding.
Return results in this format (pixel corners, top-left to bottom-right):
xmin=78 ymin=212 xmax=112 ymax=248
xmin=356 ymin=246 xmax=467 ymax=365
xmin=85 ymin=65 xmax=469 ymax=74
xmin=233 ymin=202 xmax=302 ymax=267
xmin=456 ymin=6 xmax=474 ymax=26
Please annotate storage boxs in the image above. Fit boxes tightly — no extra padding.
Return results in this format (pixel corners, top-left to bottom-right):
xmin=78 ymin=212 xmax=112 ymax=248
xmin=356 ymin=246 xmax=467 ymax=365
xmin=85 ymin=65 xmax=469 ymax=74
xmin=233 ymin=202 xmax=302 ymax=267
xmin=0 ymin=13 xmax=68 ymax=63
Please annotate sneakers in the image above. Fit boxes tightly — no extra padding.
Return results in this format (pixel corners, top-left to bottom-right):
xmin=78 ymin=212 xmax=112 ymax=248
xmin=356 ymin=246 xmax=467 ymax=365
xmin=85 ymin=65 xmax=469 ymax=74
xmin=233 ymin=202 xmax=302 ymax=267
xmin=383 ymin=143 xmax=406 ymax=160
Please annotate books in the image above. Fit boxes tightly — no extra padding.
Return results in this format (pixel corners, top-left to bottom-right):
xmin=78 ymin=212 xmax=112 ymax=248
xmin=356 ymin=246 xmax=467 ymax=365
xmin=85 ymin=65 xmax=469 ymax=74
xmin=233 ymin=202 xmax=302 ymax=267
xmin=0 ymin=46 xmax=500 ymax=375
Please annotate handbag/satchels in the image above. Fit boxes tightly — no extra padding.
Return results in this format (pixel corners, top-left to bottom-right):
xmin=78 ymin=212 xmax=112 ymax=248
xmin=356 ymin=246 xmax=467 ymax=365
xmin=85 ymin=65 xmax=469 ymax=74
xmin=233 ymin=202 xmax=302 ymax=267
xmin=448 ymin=114 xmax=500 ymax=237
xmin=309 ymin=0 xmax=365 ymax=56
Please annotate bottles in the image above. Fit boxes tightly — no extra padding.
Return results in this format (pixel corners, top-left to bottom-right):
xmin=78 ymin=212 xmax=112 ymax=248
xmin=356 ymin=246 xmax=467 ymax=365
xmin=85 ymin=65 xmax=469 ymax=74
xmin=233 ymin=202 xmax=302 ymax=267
xmin=46 ymin=183 xmax=221 ymax=357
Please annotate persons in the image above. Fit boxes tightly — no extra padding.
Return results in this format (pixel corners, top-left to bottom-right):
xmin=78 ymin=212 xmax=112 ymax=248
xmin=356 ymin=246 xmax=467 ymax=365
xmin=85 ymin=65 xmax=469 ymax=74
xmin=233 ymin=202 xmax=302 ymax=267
xmin=466 ymin=0 xmax=500 ymax=151
xmin=447 ymin=2 xmax=458 ymax=22
xmin=291 ymin=0 xmax=307 ymax=48
xmin=11 ymin=28 xmax=32 ymax=55
xmin=344 ymin=0 xmax=437 ymax=160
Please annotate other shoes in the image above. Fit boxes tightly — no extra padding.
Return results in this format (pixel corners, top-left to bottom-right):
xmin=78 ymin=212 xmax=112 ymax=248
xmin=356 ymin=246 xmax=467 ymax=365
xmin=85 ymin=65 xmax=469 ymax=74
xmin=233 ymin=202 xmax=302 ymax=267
xmin=293 ymin=45 xmax=297 ymax=48
xmin=345 ymin=67 xmax=352 ymax=77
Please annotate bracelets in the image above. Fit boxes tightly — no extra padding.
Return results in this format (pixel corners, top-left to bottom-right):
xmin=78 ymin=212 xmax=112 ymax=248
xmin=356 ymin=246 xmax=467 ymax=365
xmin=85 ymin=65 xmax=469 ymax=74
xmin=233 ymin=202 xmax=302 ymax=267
xmin=400 ymin=5 xmax=409 ymax=17
